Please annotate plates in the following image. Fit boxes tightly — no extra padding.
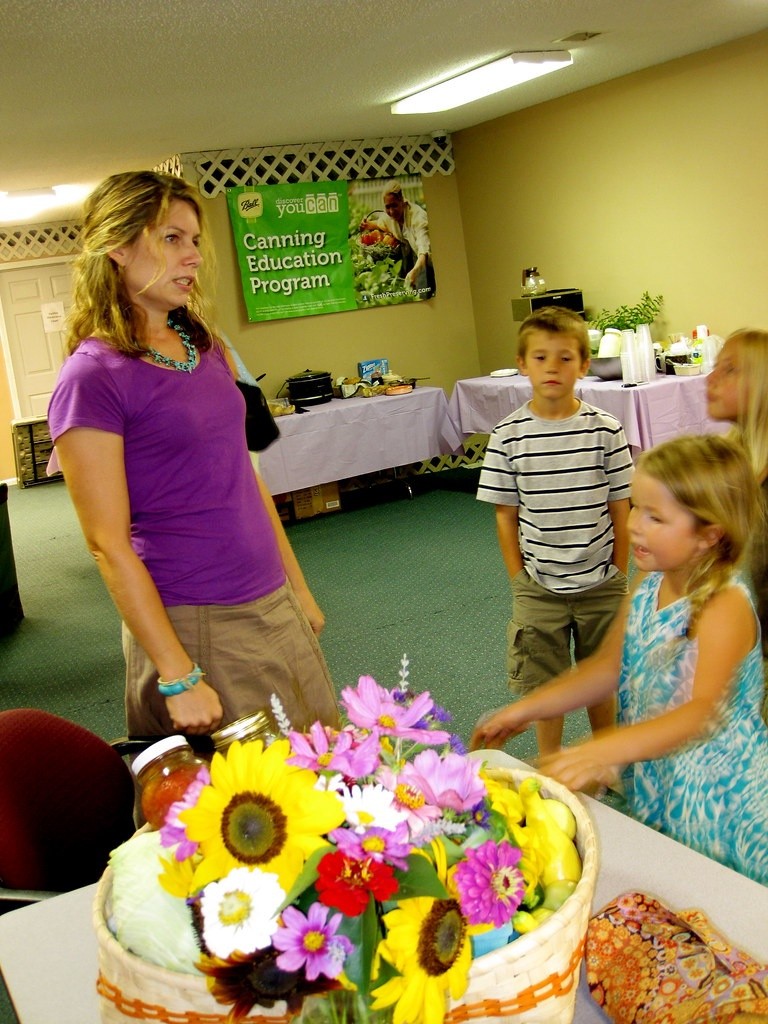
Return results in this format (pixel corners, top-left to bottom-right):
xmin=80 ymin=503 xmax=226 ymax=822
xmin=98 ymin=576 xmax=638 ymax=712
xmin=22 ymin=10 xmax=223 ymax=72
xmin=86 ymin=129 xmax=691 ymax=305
xmin=491 ymin=368 xmax=518 ymax=377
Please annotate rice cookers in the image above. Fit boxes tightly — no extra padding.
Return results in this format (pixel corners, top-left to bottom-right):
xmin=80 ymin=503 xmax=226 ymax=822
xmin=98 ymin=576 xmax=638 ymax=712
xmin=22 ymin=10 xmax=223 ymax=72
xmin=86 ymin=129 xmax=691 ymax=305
xmin=286 ymin=369 xmax=334 ymax=407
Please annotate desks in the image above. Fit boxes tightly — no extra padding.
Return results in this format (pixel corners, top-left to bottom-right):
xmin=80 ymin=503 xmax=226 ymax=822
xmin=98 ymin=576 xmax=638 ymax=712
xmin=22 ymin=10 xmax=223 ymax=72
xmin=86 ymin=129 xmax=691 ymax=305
xmin=255 ymin=384 xmax=466 ymax=525
xmin=1 ymin=748 xmax=767 ymax=1024
xmin=441 ymin=368 xmax=731 ymax=466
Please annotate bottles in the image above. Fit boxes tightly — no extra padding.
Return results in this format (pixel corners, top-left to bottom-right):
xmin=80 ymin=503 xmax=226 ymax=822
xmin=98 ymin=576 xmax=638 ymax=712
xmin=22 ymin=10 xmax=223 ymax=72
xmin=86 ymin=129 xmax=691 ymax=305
xmin=371 ymin=368 xmax=383 ymax=386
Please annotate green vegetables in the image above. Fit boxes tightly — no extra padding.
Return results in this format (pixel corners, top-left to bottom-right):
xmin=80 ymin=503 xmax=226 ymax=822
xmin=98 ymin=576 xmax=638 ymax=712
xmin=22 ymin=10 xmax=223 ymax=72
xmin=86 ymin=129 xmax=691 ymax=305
xmin=348 ymin=198 xmax=426 ymax=308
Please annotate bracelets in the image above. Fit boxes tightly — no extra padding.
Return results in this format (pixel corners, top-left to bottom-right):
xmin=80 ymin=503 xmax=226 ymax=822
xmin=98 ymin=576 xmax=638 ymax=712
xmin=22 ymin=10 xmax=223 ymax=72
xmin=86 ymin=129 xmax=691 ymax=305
xmin=158 ymin=661 xmax=204 ymax=698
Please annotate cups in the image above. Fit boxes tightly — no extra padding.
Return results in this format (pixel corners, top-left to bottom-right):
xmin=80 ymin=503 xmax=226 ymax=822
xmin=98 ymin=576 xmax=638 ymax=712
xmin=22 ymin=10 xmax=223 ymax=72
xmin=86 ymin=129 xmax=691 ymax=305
xmin=621 ymin=323 xmax=656 ymax=387
xmin=701 ymin=339 xmax=718 ymax=374
xmin=656 ymin=353 xmax=667 ymax=374
xmin=668 ymin=333 xmax=685 ymax=344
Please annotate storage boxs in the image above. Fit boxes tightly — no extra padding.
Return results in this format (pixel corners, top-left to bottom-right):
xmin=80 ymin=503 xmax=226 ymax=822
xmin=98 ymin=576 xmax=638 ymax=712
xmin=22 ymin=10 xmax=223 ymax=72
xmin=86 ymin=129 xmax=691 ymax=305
xmin=274 ymin=482 xmax=341 ymax=523
xmin=511 ymin=289 xmax=586 ymax=323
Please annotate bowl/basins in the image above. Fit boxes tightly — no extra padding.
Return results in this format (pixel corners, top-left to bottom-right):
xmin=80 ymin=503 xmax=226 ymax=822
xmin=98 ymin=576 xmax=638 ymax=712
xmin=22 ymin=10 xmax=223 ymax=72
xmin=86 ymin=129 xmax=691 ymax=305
xmin=381 ymin=370 xmax=401 ymax=384
xmin=590 ymin=357 xmax=622 ymax=380
xmin=389 ymin=377 xmax=417 ymax=390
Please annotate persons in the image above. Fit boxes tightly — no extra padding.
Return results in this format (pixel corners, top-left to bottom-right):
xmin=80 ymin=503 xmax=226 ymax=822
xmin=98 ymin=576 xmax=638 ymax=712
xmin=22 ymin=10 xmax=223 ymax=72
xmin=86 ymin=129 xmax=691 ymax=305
xmin=474 ymin=307 xmax=626 ymax=802
xmin=47 ymin=170 xmax=341 ymax=828
xmin=705 ymin=328 xmax=768 ymax=734
xmin=469 ymin=433 xmax=768 ymax=888
xmin=359 ymin=179 xmax=437 ymax=299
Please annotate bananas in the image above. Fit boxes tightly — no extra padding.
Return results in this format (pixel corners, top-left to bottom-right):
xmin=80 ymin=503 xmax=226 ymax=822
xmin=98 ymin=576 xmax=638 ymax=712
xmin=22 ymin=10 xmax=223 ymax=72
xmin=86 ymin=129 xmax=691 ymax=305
xmin=345 ymin=377 xmax=388 ymax=396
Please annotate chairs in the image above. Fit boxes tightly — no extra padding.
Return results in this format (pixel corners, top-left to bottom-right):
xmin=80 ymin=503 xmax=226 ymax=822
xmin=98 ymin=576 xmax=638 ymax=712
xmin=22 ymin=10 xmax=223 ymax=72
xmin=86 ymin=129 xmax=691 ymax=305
xmin=0 ymin=706 xmax=213 ymax=908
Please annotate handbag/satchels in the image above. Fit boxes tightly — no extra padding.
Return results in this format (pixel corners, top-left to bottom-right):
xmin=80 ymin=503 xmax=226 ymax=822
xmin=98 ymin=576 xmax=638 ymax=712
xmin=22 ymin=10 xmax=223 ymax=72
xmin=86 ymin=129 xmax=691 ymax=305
xmin=235 ymin=379 xmax=281 ymax=452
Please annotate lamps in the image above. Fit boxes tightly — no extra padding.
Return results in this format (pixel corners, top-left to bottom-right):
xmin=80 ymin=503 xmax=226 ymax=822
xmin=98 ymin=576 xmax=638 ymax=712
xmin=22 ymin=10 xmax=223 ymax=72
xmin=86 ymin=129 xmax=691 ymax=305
xmin=391 ymin=51 xmax=573 ymax=115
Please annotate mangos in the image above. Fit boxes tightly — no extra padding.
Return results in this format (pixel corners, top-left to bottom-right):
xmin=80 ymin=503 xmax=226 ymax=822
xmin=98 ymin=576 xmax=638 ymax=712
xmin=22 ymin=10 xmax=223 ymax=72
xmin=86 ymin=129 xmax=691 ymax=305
xmin=268 ymin=399 xmax=296 ymax=416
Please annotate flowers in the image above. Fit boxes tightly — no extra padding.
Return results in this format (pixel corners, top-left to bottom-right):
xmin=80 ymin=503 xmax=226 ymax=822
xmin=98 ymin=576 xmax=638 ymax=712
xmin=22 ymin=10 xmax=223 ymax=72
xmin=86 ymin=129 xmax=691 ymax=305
xmin=160 ymin=654 xmax=527 ymax=1024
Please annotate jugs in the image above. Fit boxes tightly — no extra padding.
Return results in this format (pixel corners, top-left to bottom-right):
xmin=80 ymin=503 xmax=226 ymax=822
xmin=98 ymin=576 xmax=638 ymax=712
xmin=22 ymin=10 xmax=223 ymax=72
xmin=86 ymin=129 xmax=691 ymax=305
xmin=520 ymin=267 xmax=546 ymax=297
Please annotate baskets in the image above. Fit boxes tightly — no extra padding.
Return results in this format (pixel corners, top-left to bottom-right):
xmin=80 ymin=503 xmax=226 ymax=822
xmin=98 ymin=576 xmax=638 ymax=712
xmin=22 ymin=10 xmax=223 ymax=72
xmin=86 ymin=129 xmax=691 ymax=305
xmin=356 ymin=210 xmax=401 ymax=260
xmin=92 ymin=768 xmax=598 ymax=1024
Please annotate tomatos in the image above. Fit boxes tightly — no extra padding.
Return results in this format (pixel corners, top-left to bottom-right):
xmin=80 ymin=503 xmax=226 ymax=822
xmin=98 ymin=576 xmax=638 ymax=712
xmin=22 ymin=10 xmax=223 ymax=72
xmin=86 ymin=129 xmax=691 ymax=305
xmin=371 ymin=230 xmax=380 ymax=240
xmin=485 ymin=777 xmax=580 ymax=936
xmin=391 ymin=237 xmax=398 ymax=247
xmin=383 ymin=235 xmax=390 ymax=245
xmin=361 ymin=234 xmax=373 ymax=245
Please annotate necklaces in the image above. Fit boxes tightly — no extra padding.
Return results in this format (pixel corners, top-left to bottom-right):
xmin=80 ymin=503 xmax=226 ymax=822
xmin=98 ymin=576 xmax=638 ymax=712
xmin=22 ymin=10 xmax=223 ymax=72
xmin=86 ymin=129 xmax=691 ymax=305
xmin=142 ymin=317 xmax=196 ymax=374
